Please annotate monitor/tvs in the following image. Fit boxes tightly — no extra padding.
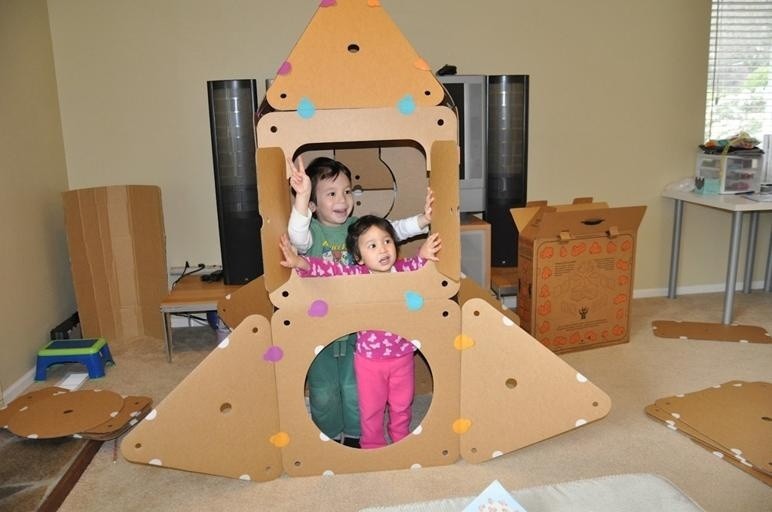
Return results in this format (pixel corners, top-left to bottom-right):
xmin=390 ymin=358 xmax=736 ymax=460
xmin=439 ymin=71 xmax=490 ymax=212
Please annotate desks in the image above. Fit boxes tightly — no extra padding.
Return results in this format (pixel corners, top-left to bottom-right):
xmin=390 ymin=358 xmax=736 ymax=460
xmin=660 ymin=191 xmax=772 ymax=325
xmin=491 ymin=267 xmax=518 ymax=300
xmin=160 ymin=276 xmax=246 ymax=363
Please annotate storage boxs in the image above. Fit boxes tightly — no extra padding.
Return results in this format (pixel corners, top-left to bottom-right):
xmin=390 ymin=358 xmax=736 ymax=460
xmin=696 ymin=154 xmax=763 ymax=195
xmin=510 ymin=196 xmax=648 ymax=355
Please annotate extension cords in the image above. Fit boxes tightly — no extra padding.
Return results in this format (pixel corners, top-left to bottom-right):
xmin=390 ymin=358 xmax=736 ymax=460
xmin=168 ymin=261 xmax=225 ymax=279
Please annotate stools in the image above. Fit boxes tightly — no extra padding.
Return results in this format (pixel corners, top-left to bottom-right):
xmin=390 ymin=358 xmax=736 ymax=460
xmin=34 ymin=337 xmax=116 ymax=383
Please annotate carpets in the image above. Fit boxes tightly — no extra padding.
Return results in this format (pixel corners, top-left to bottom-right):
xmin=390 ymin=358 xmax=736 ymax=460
xmin=0 ymin=436 xmax=87 ymax=511
xmin=358 ymin=472 xmax=707 ymax=512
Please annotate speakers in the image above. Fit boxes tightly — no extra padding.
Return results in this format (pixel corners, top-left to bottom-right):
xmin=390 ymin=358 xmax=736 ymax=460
xmin=207 ymin=78 xmax=266 ymax=287
xmin=487 ymin=67 xmax=530 ymax=269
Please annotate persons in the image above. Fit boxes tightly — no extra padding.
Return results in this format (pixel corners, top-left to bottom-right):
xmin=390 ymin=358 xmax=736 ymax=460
xmin=284 ymin=156 xmax=437 ymax=450
xmin=279 ymin=214 xmax=443 ymax=450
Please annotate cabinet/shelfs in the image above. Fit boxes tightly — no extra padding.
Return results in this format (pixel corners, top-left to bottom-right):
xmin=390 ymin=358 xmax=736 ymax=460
xmin=461 ymin=215 xmax=491 ymax=289
